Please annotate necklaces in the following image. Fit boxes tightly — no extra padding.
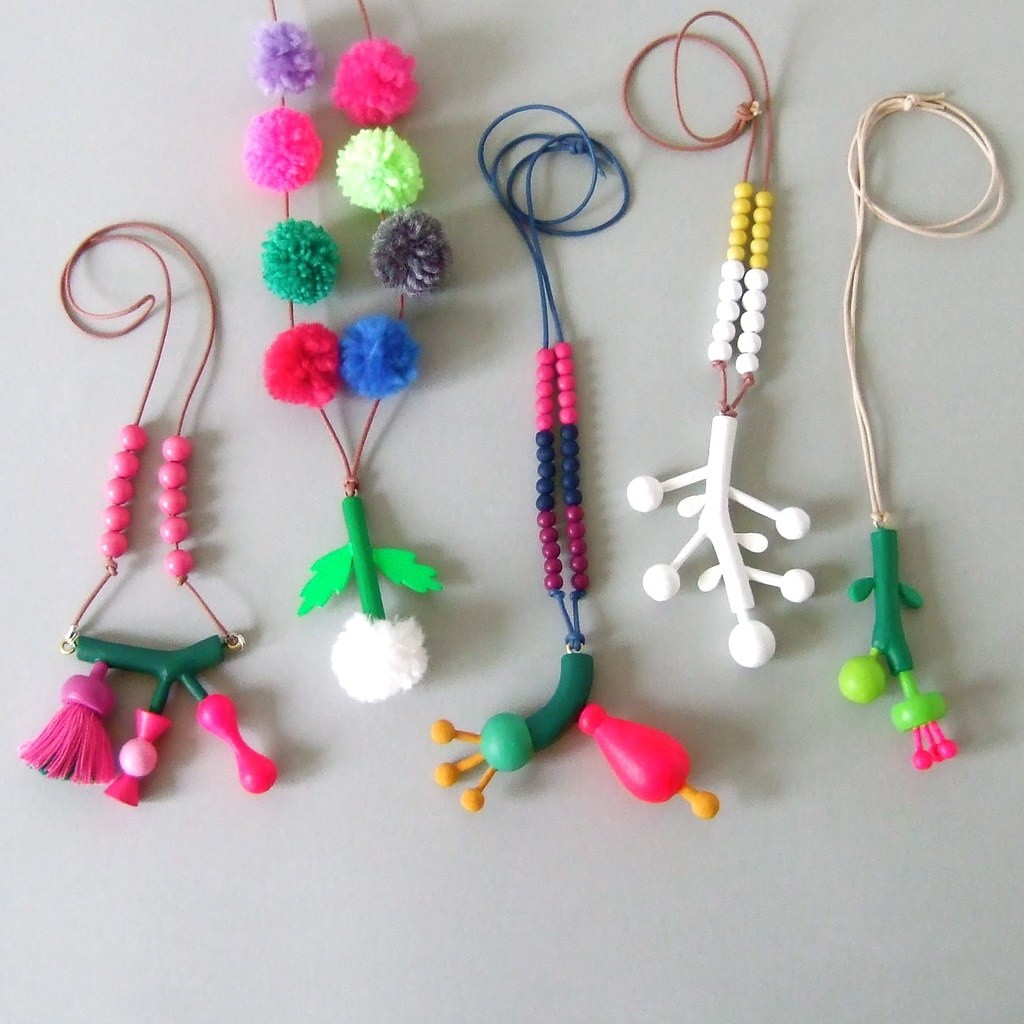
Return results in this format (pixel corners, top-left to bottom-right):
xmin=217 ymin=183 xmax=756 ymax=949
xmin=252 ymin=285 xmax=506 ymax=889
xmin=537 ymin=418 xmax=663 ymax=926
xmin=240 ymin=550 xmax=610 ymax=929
xmin=244 ymin=1 xmax=454 ymax=703
xmin=428 ymin=102 xmax=719 ymax=828
xmin=623 ymin=10 xmax=816 ymax=667
xmin=837 ymin=90 xmax=1007 ymax=770
xmin=20 ymin=219 xmax=280 ymax=809
